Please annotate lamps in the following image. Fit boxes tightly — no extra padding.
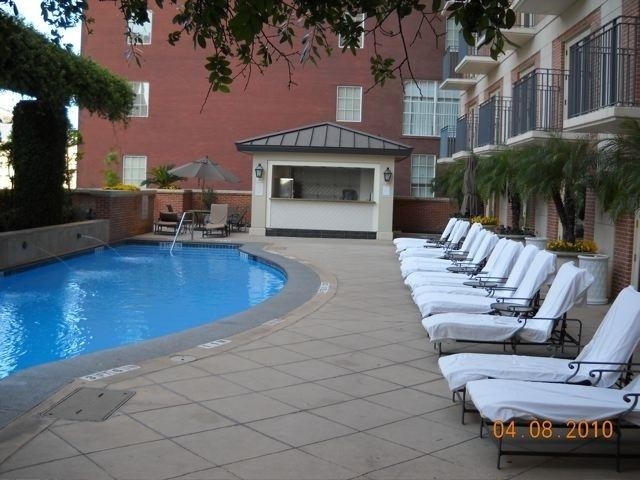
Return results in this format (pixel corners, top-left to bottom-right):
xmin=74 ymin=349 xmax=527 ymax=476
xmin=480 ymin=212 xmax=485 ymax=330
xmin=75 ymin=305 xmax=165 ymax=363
xmin=383 ymin=167 xmax=392 ymax=182
xmin=255 ymin=164 xmax=264 ymax=178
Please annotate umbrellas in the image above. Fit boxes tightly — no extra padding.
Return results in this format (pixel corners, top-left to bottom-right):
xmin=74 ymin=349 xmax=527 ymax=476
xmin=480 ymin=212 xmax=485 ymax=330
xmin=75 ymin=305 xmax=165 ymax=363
xmin=167 ymin=155 xmax=242 ymax=226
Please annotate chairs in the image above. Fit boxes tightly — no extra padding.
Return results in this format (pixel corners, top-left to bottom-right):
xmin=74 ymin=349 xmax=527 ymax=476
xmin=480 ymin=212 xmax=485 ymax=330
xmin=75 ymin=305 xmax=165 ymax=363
xmin=154 ymin=204 xmax=247 ymax=238
